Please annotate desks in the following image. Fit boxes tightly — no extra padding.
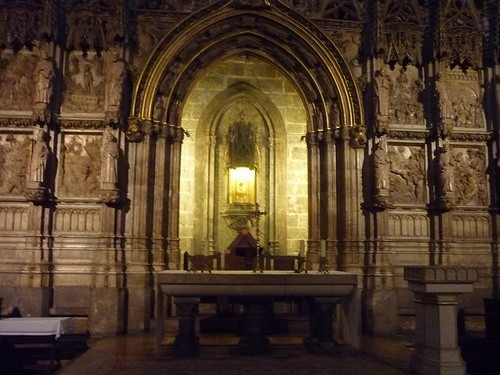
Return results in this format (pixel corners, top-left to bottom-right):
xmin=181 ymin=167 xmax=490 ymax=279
xmin=158 ymin=270 xmax=358 ymax=354
xmin=0 ymin=317 xmax=87 ymax=373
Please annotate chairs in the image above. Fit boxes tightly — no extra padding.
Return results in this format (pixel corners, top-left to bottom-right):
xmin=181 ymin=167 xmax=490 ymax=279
xmin=183 ymin=250 xmax=305 ymax=272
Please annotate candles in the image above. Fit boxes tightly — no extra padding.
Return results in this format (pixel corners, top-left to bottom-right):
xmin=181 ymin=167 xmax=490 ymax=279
xmin=319 ymin=239 xmax=327 ymax=257
xmin=299 ymin=239 xmax=306 ymax=258
